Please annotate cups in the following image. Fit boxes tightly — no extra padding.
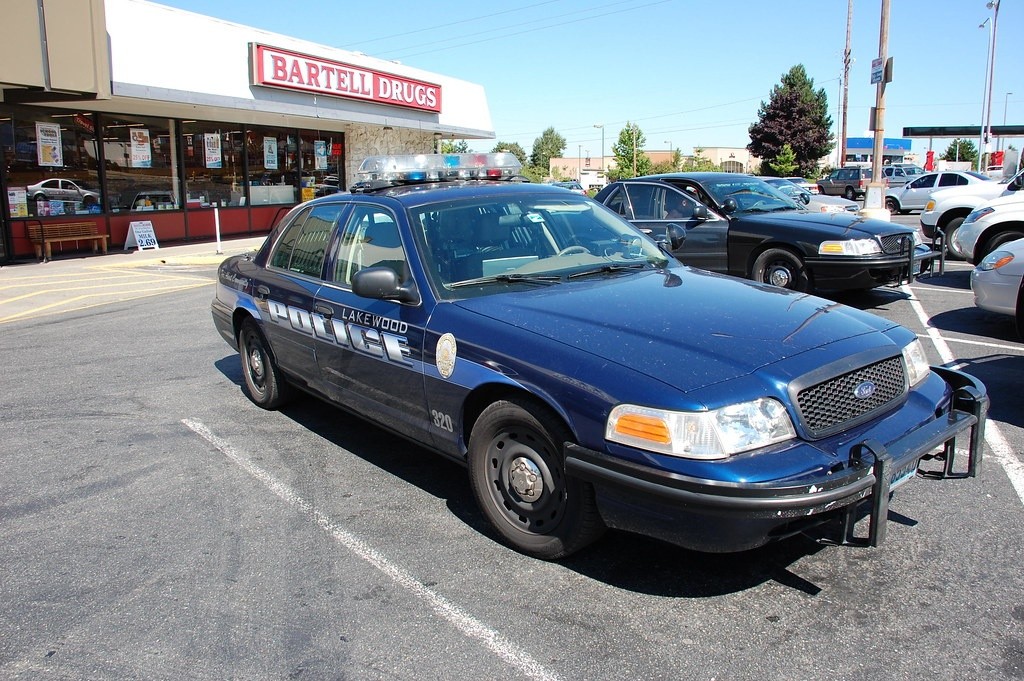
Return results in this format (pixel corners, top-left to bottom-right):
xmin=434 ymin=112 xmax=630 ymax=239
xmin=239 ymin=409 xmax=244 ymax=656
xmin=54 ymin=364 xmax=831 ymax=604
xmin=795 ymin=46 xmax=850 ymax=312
xmin=137 ymin=206 xmax=142 ymax=210
xmin=174 ymin=204 xmax=179 ymax=209
xmin=221 ymin=199 xmax=227 ymax=208
xmin=166 ymin=204 xmax=173 ymax=209
xmin=158 ymin=204 xmax=165 ymax=209
xmin=249 ymin=180 xmax=252 ymax=186
xmin=213 ymin=201 xmax=217 ymax=207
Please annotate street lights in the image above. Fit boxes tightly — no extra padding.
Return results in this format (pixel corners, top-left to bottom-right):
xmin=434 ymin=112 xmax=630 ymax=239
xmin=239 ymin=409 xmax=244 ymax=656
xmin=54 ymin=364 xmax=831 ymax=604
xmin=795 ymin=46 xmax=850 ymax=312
xmin=1002 ymin=93 xmax=1012 ymax=151
xmin=664 ymin=141 xmax=672 ymax=162
xmin=984 ymin=0 xmax=1001 ymax=178
xmin=956 ymin=140 xmax=961 ymax=162
xmin=978 ymin=17 xmax=992 ymax=173
xmin=578 ymin=145 xmax=582 ymax=182
xmin=541 ymin=153 xmax=545 ymax=178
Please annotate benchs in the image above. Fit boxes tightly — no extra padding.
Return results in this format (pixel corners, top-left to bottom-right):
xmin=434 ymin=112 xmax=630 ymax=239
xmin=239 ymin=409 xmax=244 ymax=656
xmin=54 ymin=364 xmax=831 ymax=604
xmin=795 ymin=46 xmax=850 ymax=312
xmin=27 ymin=221 xmax=110 ymax=261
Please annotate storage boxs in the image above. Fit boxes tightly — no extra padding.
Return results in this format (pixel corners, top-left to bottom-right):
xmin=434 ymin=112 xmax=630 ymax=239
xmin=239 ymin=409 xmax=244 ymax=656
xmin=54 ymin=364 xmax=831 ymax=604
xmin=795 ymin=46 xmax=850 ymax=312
xmin=187 ymin=199 xmax=201 ymax=209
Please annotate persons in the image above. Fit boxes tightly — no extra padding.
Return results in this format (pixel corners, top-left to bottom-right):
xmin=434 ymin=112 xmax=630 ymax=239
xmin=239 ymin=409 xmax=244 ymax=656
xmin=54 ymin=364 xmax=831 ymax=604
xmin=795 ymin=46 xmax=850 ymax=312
xmin=665 ymin=191 xmax=697 ymax=219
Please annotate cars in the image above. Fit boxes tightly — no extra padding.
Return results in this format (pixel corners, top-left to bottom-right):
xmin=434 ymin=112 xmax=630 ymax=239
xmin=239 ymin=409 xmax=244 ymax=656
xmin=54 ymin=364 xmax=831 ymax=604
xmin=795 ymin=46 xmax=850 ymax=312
xmin=552 ymin=182 xmax=587 ymax=195
xmin=716 ymin=176 xmax=860 ymax=214
xmin=884 ymin=171 xmax=1009 ymax=216
xmin=882 ymin=164 xmax=927 ymax=188
xmin=8 ymin=153 xmax=339 ymax=209
xmin=210 ymin=152 xmax=990 ymax=561
xmin=779 ymin=178 xmax=819 ymax=195
xmin=970 ymin=238 xmax=1024 ymax=339
xmin=920 ymin=168 xmax=1024 ymax=261
xmin=508 ymin=172 xmax=932 ymax=291
xmin=956 ymin=194 xmax=1024 ymax=268
xmin=981 ymin=166 xmax=1003 ymax=177
xmin=817 ymin=165 xmax=889 ymax=201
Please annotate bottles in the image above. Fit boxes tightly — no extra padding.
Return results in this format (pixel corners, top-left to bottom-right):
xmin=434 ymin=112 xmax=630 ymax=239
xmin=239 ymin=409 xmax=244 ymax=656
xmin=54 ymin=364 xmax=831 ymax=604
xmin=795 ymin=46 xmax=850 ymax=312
xmin=199 ymin=191 xmax=209 ymax=208
xmin=145 ymin=196 xmax=152 ymax=206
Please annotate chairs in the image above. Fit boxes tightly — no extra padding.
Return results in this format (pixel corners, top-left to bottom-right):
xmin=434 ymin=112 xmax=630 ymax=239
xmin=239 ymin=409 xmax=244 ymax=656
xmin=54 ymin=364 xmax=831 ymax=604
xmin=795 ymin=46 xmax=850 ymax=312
xmin=364 ymin=222 xmax=412 ymax=287
xmin=456 ymin=214 xmax=540 ymax=281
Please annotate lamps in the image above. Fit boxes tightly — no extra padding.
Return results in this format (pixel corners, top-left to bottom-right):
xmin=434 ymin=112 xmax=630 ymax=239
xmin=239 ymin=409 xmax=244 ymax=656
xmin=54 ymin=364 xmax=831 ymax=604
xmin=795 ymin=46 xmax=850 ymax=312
xmin=383 ymin=127 xmax=393 ymax=133
xmin=434 ymin=132 xmax=443 ymax=140
